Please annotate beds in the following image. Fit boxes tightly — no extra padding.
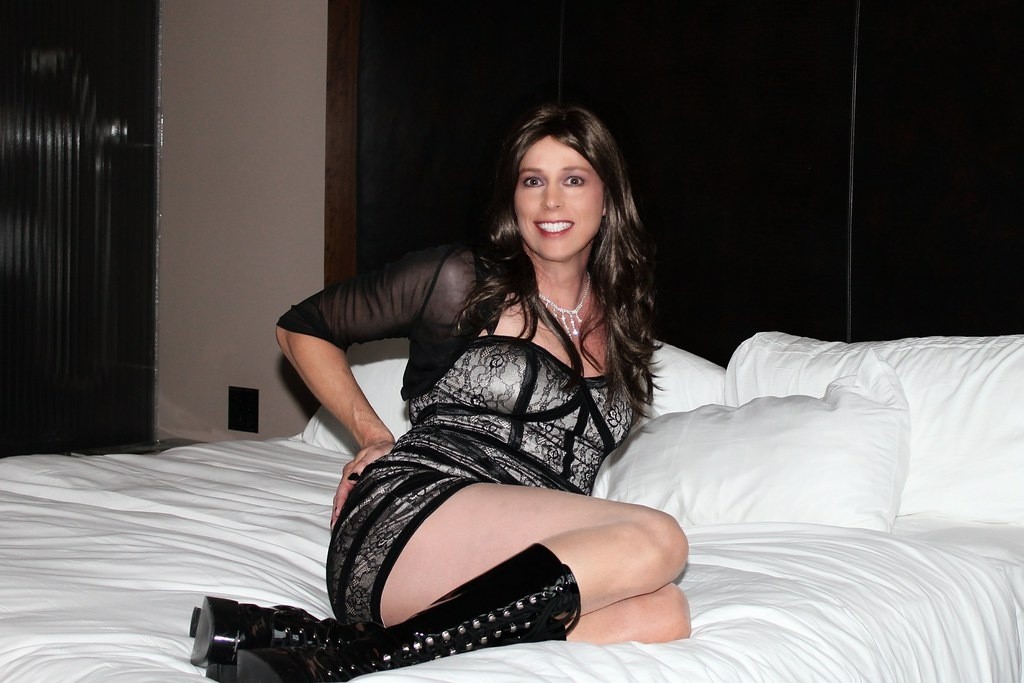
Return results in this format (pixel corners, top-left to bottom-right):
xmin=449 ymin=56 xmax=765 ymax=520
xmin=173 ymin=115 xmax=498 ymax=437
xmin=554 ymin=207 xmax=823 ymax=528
xmin=0 ymin=328 xmax=1023 ymax=683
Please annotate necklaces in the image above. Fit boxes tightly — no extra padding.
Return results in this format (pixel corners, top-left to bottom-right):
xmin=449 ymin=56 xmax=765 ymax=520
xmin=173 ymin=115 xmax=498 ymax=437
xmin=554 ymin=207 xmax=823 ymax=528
xmin=534 ymin=268 xmax=591 ymax=340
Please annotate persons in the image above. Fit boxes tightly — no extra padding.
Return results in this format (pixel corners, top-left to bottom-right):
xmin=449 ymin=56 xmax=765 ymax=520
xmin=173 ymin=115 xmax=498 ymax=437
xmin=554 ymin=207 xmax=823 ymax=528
xmin=187 ymin=103 xmax=693 ymax=683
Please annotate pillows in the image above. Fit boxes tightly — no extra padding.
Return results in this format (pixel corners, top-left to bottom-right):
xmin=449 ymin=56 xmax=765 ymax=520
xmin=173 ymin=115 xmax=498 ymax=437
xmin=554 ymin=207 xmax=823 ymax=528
xmin=584 ymin=347 xmax=913 ymax=537
xmin=724 ymin=331 xmax=1024 ymax=529
xmin=301 ymin=357 xmax=412 ymax=456
xmin=639 ymin=338 xmax=737 ymax=420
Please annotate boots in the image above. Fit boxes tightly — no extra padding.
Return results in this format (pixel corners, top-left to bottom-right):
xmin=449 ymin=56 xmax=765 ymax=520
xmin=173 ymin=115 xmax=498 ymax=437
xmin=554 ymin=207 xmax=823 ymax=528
xmin=191 ymin=543 xmax=581 ymax=683
